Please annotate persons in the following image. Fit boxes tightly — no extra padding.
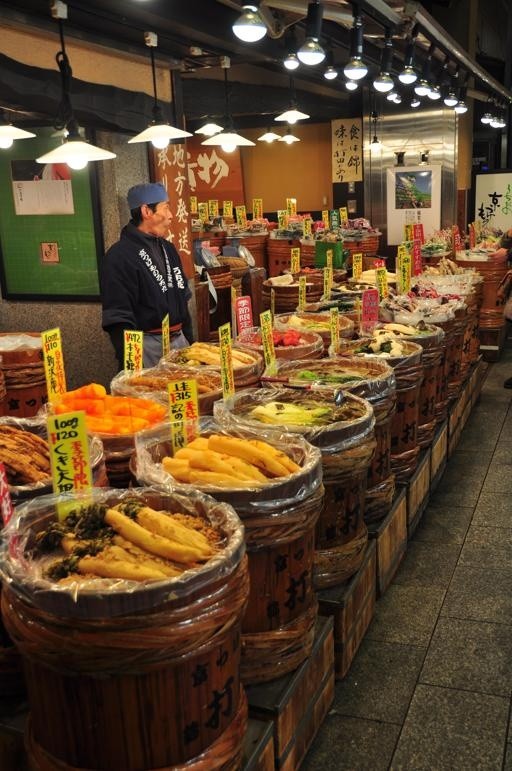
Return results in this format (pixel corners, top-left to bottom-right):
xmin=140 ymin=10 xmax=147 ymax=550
xmin=487 ymin=224 xmax=512 ymax=389
xmin=100 ymin=182 xmax=193 ymax=369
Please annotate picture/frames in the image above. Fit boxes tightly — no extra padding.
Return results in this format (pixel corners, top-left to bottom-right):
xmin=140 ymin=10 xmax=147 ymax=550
xmin=0 ymin=120 xmax=105 ymax=303
xmin=470 ymin=166 xmax=512 ymax=239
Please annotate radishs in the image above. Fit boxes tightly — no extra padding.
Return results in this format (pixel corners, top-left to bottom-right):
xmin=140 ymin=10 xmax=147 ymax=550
xmin=60 ymin=504 xmax=229 ymax=584
xmin=162 ymin=434 xmax=302 ymax=487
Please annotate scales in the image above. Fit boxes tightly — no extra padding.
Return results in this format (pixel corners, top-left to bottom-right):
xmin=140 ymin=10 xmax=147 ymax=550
xmin=223 ymin=236 xmax=256 ymax=268
xmin=193 ymin=239 xmax=221 ymax=269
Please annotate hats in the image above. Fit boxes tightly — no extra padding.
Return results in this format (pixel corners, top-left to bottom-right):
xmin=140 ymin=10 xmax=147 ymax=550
xmin=127 ymin=183 xmax=167 ymax=210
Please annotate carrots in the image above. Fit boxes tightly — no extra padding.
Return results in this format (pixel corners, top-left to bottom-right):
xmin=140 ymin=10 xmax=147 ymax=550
xmin=52 ymin=383 xmax=168 ymax=436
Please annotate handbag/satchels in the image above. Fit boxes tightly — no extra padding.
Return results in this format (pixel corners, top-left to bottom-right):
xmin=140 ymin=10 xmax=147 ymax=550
xmin=497 ymin=271 xmax=512 ymax=299
